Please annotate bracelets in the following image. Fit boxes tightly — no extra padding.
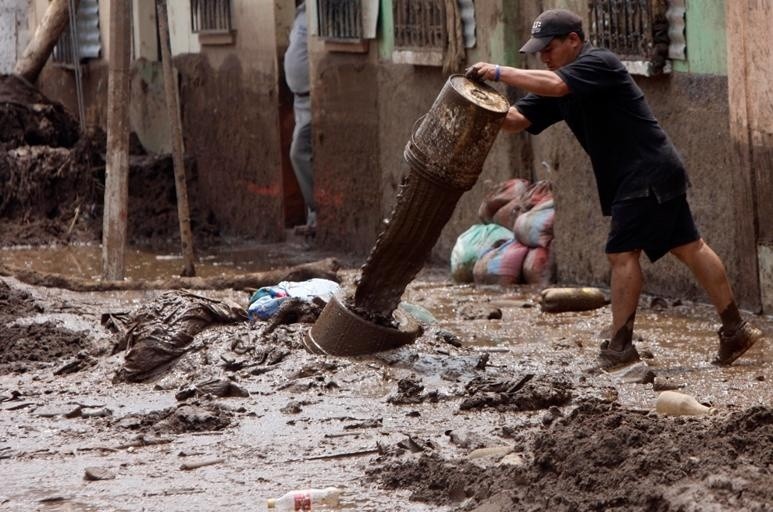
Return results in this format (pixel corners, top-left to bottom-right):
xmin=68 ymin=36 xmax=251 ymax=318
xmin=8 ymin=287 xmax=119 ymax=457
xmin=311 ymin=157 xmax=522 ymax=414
xmin=494 ymin=63 xmax=500 ymax=81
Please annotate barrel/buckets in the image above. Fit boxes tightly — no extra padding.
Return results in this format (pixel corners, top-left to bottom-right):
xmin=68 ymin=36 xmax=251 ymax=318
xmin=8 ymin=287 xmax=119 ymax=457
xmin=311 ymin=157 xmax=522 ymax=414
xmin=403 ymin=74 xmax=511 ymax=192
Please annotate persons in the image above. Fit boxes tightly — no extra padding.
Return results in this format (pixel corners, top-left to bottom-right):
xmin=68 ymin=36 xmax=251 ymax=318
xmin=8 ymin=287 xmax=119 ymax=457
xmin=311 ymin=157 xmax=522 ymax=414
xmin=282 ymin=1 xmax=316 ymax=229
xmin=466 ymin=10 xmax=761 ymax=371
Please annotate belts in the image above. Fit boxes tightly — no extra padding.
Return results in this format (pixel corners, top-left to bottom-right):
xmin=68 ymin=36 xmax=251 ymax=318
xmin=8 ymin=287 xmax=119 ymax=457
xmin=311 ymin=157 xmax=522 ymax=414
xmin=295 ymin=91 xmax=309 ymax=97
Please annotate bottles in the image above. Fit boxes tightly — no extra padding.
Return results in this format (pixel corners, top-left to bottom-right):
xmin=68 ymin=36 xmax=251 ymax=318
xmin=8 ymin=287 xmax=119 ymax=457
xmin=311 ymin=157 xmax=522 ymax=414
xmin=265 ymin=485 xmax=339 ymax=512
xmin=655 ymin=390 xmax=717 ymax=416
xmin=246 ymin=294 xmax=272 ymax=321
xmin=537 ymin=287 xmax=604 ymax=313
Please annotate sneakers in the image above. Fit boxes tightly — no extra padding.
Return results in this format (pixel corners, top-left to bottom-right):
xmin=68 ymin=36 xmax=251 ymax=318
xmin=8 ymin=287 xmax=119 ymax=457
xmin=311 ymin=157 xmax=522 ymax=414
xmin=709 ymin=319 xmax=762 ymax=367
xmin=587 ymin=340 xmax=643 ymax=375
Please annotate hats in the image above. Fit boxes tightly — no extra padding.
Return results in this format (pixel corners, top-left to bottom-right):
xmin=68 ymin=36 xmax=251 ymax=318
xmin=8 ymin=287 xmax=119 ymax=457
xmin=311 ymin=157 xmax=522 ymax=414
xmin=518 ymin=8 xmax=585 ymax=55
